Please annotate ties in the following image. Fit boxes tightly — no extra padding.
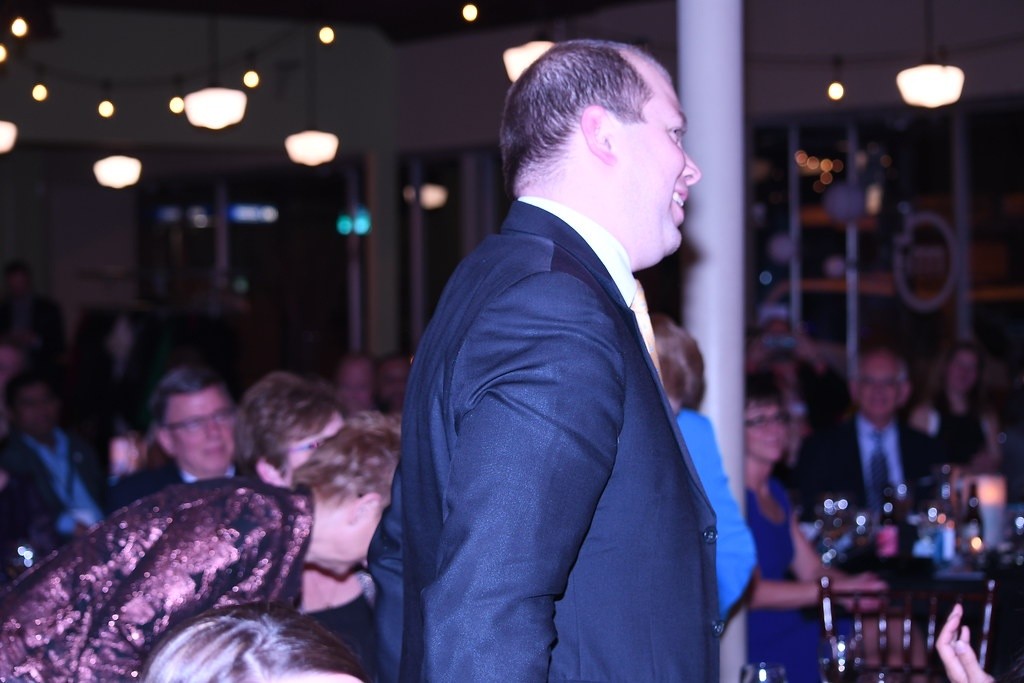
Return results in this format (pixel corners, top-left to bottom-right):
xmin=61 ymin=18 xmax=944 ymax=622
xmin=869 ymin=431 xmax=889 ymax=516
xmin=631 ymin=279 xmax=664 ymax=388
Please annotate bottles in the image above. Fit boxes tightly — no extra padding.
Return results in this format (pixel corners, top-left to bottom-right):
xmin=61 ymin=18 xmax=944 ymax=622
xmin=880 ymin=466 xmax=984 ymax=564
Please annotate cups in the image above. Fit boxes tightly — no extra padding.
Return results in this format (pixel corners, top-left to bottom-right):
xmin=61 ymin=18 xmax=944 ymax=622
xmin=738 ymin=662 xmax=788 ymax=683
xmin=817 ymin=634 xmax=864 ymax=683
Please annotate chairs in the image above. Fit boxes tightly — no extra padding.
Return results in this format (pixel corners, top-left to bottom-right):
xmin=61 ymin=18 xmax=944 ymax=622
xmin=817 ymin=575 xmax=999 ymax=683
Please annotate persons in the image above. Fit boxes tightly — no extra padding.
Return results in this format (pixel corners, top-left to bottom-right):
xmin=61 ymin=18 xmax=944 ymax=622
xmin=367 ymin=36 xmax=726 ymax=682
xmin=2 ymin=260 xmax=1014 ymax=680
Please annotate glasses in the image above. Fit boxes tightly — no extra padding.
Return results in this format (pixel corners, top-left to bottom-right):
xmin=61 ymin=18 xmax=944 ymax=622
xmin=855 ymin=374 xmax=904 ymax=387
xmin=286 ymin=440 xmax=325 ymax=453
xmin=166 ymin=408 xmax=230 ymax=431
xmin=744 ymin=413 xmax=787 ymax=428
